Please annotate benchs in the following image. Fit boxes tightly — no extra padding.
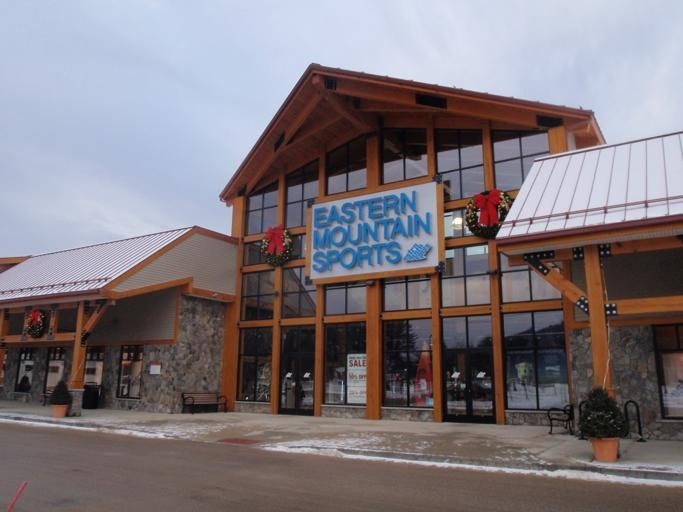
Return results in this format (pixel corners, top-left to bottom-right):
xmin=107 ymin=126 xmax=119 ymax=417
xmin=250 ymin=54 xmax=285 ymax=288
xmin=180 ymin=392 xmax=227 ymax=415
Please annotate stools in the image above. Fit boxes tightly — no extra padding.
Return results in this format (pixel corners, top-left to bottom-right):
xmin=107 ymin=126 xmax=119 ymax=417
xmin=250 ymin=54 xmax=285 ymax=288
xmin=547 ymin=403 xmax=574 ymax=434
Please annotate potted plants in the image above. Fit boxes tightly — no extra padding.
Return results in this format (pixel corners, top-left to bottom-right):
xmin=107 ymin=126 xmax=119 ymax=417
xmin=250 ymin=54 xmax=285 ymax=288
xmin=48 ymin=380 xmax=71 ymax=418
xmin=577 ymin=384 xmax=628 ymax=462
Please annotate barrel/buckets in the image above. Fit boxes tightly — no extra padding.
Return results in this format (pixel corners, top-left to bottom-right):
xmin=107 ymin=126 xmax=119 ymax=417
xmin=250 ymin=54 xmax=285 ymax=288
xmin=83 ymin=385 xmax=101 ymax=409
